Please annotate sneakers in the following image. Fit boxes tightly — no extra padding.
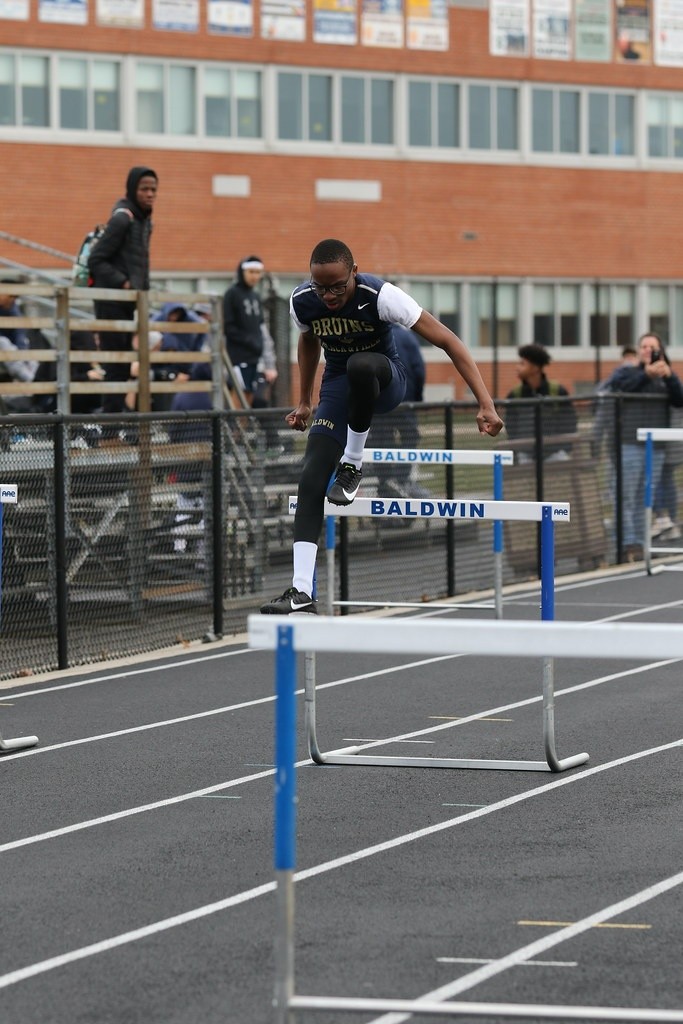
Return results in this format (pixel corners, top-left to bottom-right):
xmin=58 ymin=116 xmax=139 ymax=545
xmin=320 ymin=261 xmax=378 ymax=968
xmin=651 ymin=516 xmax=674 ymax=538
xmin=659 ymin=527 xmax=680 ymax=541
xmin=260 ymin=586 xmax=317 ymax=614
xmin=328 ymin=462 xmax=363 ymax=507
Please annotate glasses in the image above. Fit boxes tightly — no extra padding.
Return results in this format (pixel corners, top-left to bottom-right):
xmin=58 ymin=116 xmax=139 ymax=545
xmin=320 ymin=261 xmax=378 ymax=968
xmin=309 ymin=267 xmax=352 ymax=295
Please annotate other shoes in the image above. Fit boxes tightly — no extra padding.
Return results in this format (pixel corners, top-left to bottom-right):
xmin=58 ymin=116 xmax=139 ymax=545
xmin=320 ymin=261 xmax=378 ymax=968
xmin=98 ymin=438 xmax=128 ymax=447
xmin=378 ymin=481 xmax=432 ymax=499
xmin=627 ymin=544 xmax=657 ymax=562
xmin=267 ymin=446 xmax=290 ymax=455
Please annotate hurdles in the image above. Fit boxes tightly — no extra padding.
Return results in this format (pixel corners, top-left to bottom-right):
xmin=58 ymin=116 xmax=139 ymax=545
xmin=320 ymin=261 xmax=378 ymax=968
xmin=287 ymin=491 xmax=592 ymax=774
xmin=245 ymin=611 xmax=683 ymax=1023
xmin=636 ymin=424 xmax=683 ymax=577
xmin=323 ymin=440 xmax=519 ymax=621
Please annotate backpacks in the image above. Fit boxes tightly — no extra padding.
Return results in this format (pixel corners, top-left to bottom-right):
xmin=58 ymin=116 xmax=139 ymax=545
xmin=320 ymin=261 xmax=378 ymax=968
xmin=70 ymin=208 xmax=134 ymax=287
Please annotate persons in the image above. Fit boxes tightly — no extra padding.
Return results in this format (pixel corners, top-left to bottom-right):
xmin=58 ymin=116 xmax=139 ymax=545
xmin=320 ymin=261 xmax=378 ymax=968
xmin=505 ymin=344 xmax=577 ymax=461
xmin=0 ymin=167 xmax=278 ymax=573
xmin=260 ymin=239 xmax=504 ymax=614
xmin=592 ymin=333 xmax=683 ymax=563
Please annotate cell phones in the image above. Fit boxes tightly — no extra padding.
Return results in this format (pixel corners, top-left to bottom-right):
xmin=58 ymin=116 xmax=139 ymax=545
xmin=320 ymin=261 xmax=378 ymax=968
xmin=651 ymin=351 xmax=660 ymax=364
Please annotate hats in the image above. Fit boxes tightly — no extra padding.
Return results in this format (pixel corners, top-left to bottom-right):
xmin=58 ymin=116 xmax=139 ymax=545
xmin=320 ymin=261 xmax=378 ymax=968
xmin=520 ymin=346 xmax=550 ymax=367
